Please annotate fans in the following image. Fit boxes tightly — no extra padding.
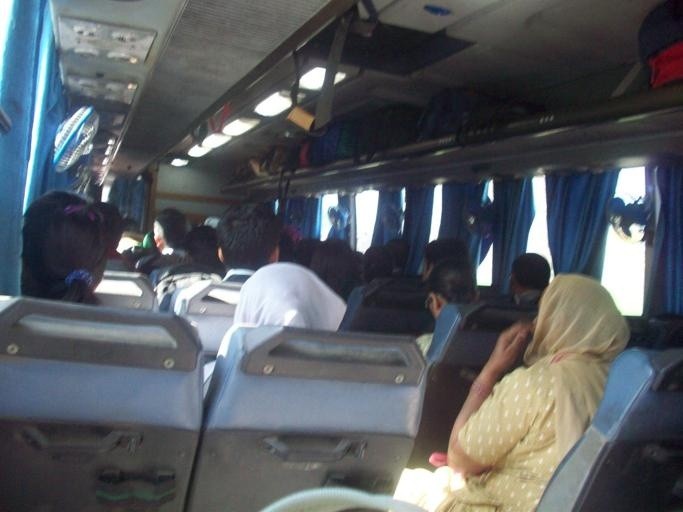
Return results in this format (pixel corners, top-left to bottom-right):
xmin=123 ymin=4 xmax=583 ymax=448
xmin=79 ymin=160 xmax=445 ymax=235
xmin=51 ymin=105 xmax=100 ymax=173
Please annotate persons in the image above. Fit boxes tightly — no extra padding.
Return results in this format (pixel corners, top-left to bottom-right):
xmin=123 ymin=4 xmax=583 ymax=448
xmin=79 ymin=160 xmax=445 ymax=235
xmin=390 ymin=271 xmax=633 ymax=512
xmin=281 ymin=232 xmax=551 ymax=355
xmin=21 ymin=193 xmax=139 ymax=305
xmin=137 ymin=199 xmax=347 ymax=398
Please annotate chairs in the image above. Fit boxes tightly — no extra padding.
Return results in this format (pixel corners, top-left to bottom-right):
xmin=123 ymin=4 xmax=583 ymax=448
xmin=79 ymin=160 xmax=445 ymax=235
xmin=0 ymin=233 xmax=681 ymax=511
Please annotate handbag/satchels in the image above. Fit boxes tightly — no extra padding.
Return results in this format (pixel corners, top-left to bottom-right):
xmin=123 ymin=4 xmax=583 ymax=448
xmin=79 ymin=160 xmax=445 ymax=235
xmin=310 ymin=82 xmax=543 ymax=169
xmin=229 ymin=135 xmax=312 ymax=183
xmin=637 ymin=1 xmax=683 ymax=90
